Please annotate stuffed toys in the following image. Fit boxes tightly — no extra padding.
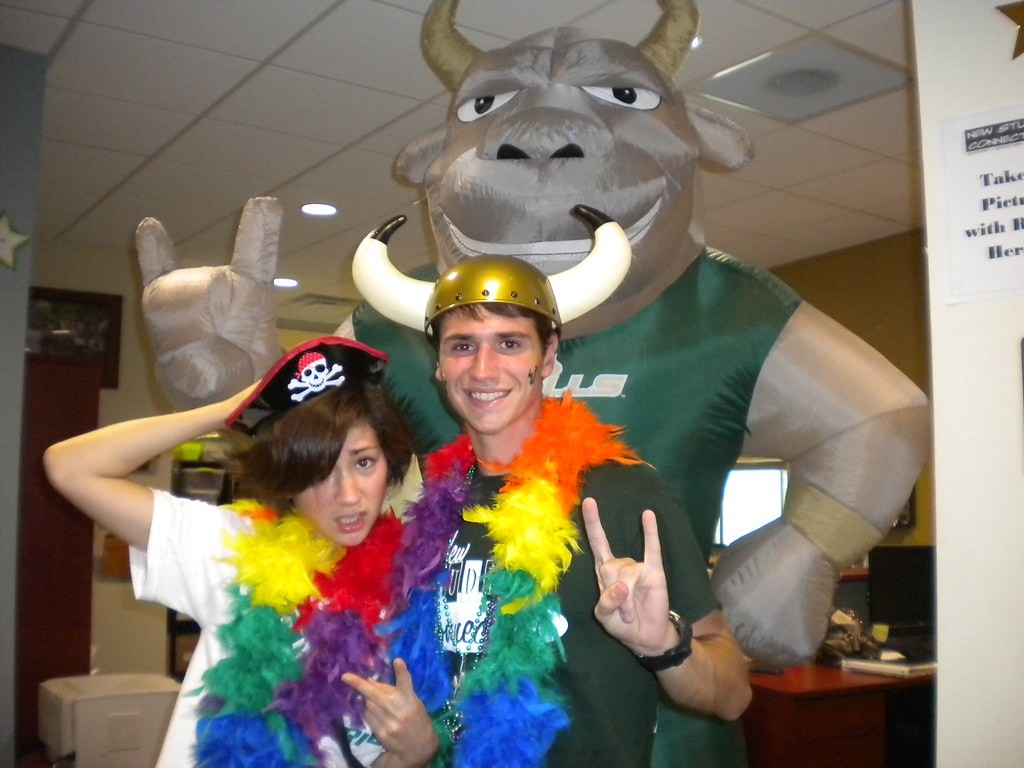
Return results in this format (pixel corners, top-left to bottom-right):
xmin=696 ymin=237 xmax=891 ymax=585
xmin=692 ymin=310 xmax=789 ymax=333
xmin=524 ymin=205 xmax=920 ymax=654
xmin=132 ymin=1 xmax=931 ymax=768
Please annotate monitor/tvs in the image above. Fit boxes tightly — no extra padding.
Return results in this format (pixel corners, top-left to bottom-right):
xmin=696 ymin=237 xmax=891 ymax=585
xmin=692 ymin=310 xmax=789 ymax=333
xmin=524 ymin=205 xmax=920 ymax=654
xmin=866 ymin=544 xmax=934 ymax=637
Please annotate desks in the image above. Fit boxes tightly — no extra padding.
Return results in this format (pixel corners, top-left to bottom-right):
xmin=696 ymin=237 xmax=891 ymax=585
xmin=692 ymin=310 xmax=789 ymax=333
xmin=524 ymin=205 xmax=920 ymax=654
xmin=751 ymin=667 xmax=936 ymax=768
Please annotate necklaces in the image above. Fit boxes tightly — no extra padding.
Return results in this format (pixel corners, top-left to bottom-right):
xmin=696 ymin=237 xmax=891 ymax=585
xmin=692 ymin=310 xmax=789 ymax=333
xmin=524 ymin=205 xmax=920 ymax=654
xmin=389 ymin=390 xmax=646 ymax=767
xmin=193 ymin=490 xmax=406 ymax=768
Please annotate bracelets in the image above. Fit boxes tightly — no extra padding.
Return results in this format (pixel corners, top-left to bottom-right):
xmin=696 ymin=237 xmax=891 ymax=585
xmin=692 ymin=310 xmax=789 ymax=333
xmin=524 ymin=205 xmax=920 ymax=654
xmin=635 ymin=610 xmax=695 ymax=675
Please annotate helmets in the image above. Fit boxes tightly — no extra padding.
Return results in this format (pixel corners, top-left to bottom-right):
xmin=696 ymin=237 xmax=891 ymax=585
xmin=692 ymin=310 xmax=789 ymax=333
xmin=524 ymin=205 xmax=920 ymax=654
xmin=424 ymin=255 xmax=562 ymax=351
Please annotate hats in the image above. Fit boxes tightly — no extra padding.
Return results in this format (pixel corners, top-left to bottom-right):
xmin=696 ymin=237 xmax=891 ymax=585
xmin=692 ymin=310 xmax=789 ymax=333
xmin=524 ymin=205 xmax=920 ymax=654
xmin=224 ymin=337 xmax=389 ymax=438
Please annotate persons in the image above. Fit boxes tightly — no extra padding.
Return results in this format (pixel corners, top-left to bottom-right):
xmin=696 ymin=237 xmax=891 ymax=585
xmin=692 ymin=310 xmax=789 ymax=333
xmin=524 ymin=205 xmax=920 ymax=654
xmin=424 ymin=252 xmax=754 ymax=768
xmin=42 ymin=334 xmax=440 ymax=768
